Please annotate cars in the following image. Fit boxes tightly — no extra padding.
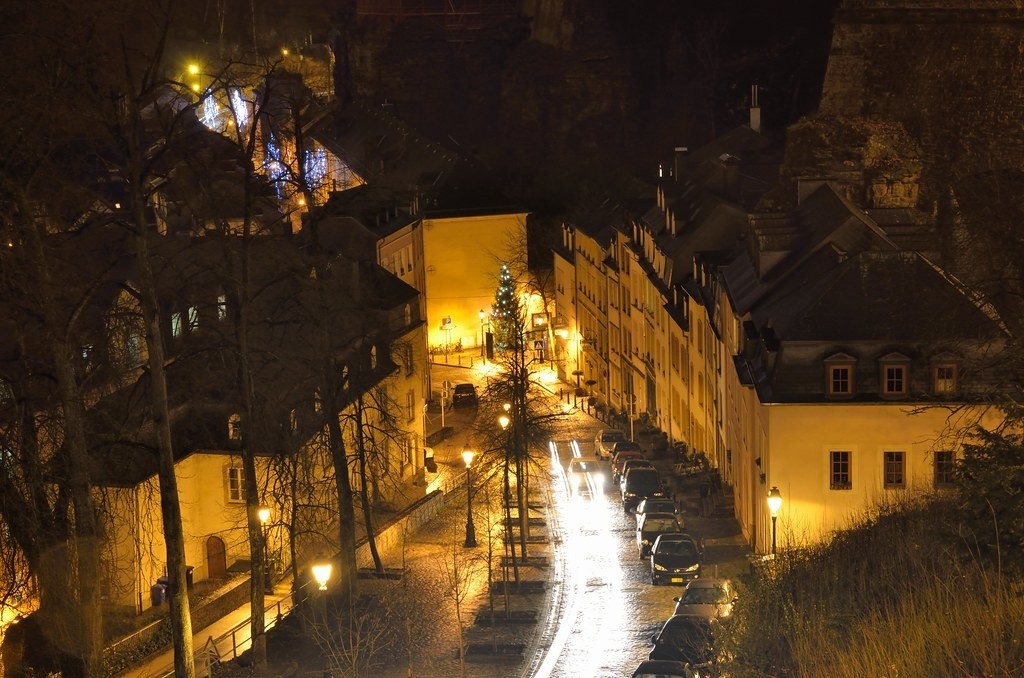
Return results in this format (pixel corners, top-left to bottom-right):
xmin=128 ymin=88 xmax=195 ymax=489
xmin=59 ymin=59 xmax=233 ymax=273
xmin=649 ymin=614 xmax=718 ymax=664
xmin=594 ymin=429 xmax=626 ymax=460
xmin=649 ymin=533 xmax=701 ymax=585
xmin=632 ymin=660 xmax=699 ymax=678
xmin=453 ymin=384 xmax=478 ymax=407
xmin=637 ymin=513 xmax=683 ymax=559
xmin=635 ymin=497 xmax=683 ymax=546
xmin=609 ymin=442 xmax=666 ymax=513
xmin=674 ymin=578 xmax=738 ymax=624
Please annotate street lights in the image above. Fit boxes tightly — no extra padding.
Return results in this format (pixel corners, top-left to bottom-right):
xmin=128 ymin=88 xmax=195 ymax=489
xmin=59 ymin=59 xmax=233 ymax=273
xmin=499 ymin=415 xmax=509 ymax=499
xmin=310 ymin=550 xmax=332 ymax=678
xmin=767 ymin=486 xmax=782 ymax=561
xmin=257 ymin=503 xmax=276 ymax=595
xmin=460 ymin=443 xmax=477 ymax=548
xmin=478 ymin=308 xmax=485 ymax=356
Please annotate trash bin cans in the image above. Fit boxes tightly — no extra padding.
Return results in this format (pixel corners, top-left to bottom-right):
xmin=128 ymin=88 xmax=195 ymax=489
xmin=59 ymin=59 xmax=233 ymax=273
xmin=186 ymin=565 xmax=194 ymax=589
xmin=151 ymin=584 xmax=165 ymax=606
xmin=157 ymin=576 xmax=169 ymax=600
